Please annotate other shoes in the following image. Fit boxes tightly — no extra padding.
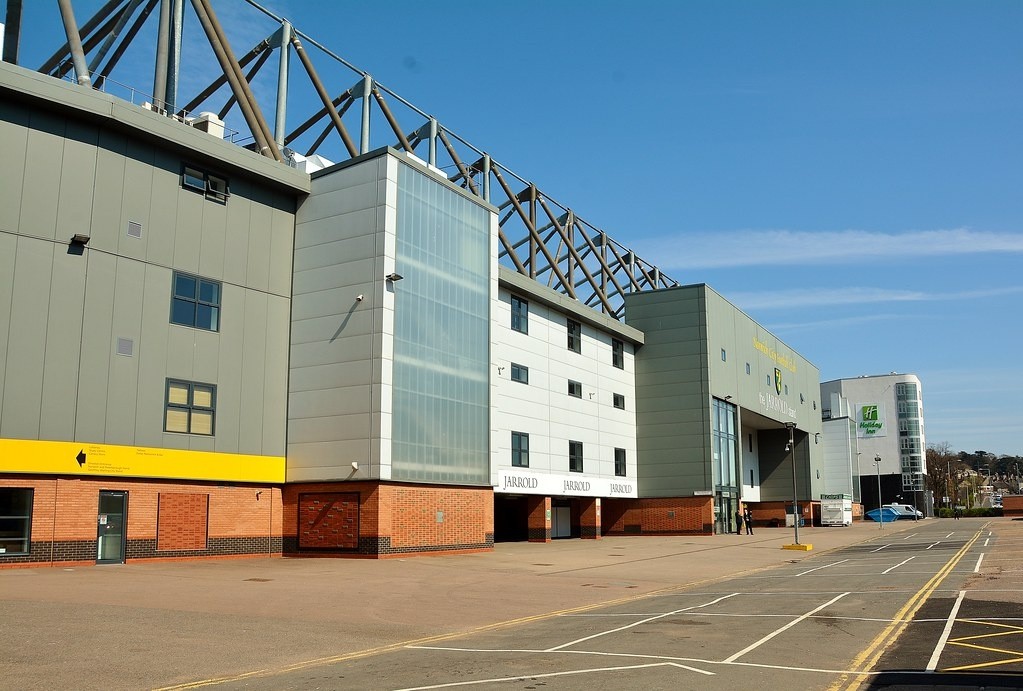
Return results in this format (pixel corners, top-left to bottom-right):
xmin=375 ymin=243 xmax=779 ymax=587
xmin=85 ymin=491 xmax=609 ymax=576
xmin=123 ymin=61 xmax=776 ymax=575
xmin=747 ymin=534 xmax=749 ymax=535
xmin=751 ymin=533 xmax=753 ymax=535
xmin=737 ymin=534 xmax=741 ymax=535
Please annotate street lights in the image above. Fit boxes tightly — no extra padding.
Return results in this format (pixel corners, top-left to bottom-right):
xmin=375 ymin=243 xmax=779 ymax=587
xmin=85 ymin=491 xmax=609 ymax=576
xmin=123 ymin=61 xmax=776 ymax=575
xmin=785 ymin=422 xmax=799 ymax=544
xmin=873 ymin=453 xmax=883 ymax=528
xmin=948 ymin=459 xmax=961 ymax=508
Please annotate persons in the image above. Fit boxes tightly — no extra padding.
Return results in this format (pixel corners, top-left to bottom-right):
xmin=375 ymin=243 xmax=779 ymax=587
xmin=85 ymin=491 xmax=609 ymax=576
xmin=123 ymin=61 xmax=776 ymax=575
xmin=744 ymin=508 xmax=754 ymax=535
xmin=960 ymin=508 xmax=963 ymax=517
xmin=955 ymin=510 xmax=959 ymax=520
xmin=735 ymin=510 xmax=743 ymax=535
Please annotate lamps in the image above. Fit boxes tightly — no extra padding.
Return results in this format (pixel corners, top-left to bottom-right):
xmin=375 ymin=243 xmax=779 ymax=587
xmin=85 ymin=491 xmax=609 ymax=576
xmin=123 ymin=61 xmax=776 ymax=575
xmin=725 ymin=395 xmax=732 ymax=399
xmin=385 ymin=273 xmax=404 ymax=281
xmin=71 ymin=233 xmax=90 ymax=245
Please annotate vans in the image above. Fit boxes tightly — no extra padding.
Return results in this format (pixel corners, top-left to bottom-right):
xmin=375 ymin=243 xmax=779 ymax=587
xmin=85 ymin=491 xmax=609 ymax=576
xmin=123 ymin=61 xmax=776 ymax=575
xmin=883 ymin=503 xmax=923 ymax=520
